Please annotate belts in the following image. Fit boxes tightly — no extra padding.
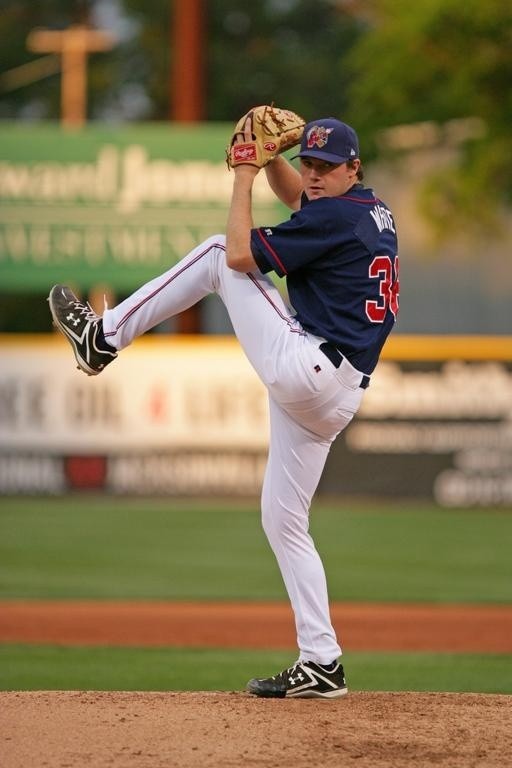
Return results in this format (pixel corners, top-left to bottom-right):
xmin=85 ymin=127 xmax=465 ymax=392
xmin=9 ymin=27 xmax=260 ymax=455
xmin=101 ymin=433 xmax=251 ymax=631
xmin=319 ymin=341 xmax=371 ymax=390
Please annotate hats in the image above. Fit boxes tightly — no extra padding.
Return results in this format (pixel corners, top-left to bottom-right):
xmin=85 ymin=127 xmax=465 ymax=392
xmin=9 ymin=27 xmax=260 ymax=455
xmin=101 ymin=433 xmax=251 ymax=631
xmin=288 ymin=114 xmax=360 ymax=164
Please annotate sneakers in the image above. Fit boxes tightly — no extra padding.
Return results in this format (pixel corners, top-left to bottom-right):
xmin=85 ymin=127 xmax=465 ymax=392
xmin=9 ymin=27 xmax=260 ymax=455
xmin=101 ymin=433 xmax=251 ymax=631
xmin=246 ymin=658 xmax=349 ymax=700
xmin=48 ymin=282 xmax=118 ymax=377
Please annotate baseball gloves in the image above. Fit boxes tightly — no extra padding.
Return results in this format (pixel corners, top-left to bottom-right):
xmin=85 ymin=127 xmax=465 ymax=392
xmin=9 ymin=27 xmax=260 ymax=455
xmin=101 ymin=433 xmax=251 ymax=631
xmin=225 ymin=104 xmax=307 ymax=168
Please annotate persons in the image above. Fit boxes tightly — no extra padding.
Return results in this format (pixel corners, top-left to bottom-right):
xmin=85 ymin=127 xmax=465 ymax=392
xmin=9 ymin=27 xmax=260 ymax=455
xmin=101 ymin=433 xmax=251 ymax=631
xmin=47 ymin=106 xmax=406 ymax=701
